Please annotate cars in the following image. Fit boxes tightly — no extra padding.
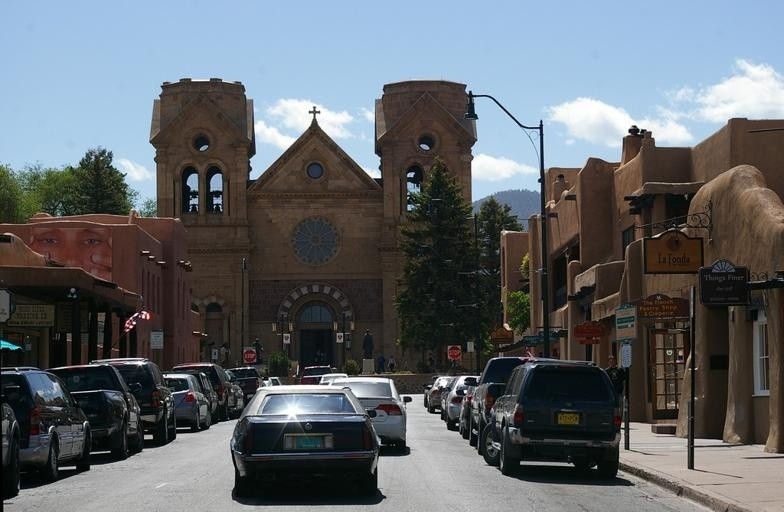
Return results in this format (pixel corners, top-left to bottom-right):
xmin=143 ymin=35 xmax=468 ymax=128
xmin=0 ymin=393 xmax=23 ymax=498
xmin=326 ymin=376 xmax=413 ymax=452
xmin=230 ymin=385 xmax=381 ymax=496
xmin=171 ymin=361 xmax=282 ymax=422
xmin=318 ymin=372 xmax=348 ymax=385
xmin=423 ymin=373 xmax=479 ymax=439
xmin=161 ymin=374 xmax=211 ymax=431
xmin=161 ymin=369 xmax=219 ymax=424
xmin=300 ymin=366 xmax=333 ymax=384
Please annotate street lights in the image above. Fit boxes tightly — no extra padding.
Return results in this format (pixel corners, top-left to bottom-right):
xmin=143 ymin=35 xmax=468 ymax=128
xmin=462 ymin=90 xmax=551 ymax=355
xmin=417 ymin=243 xmax=458 ymax=342
xmin=427 ymin=197 xmax=482 ymax=373
xmin=240 ymin=256 xmax=247 ymax=355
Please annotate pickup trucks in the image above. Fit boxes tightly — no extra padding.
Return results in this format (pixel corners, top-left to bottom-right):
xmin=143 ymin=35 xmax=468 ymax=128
xmin=464 ymin=357 xmax=561 ymax=453
xmin=42 ymin=364 xmax=144 ymax=461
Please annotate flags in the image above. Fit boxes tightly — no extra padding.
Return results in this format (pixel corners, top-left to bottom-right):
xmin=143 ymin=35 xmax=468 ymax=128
xmin=123 ymin=311 xmax=151 ymax=332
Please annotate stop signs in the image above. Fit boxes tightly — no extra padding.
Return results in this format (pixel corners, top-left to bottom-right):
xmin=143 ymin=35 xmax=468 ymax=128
xmin=446 ymin=345 xmax=462 ymax=361
xmin=242 ymin=347 xmax=257 ymax=364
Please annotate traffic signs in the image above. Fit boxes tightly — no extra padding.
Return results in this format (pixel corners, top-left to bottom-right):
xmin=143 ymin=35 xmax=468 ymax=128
xmin=613 ymin=301 xmax=641 ymax=342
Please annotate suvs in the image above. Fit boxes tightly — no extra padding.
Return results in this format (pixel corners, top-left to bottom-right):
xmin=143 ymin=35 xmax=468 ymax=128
xmin=480 ymin=360 xmax=622 ymax=480
xmin=88 ymin=358 xmax=176 ymax=444
xmin=0 ymin=366 xmax=93 ymax=480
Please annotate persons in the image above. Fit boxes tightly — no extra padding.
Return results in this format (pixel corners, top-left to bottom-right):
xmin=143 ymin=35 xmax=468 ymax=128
xmin=362 ymin=328 xmax=374 ymax=359
xmin=29 ymin=228 xmax=112 ymax=282
xmin=387 ymin=355 xmax=397 ymax=372
xmin=603 ymin=354 xmax=625 ymax=398
xmin=377 ymin=354 xmax=386 ymax=372
